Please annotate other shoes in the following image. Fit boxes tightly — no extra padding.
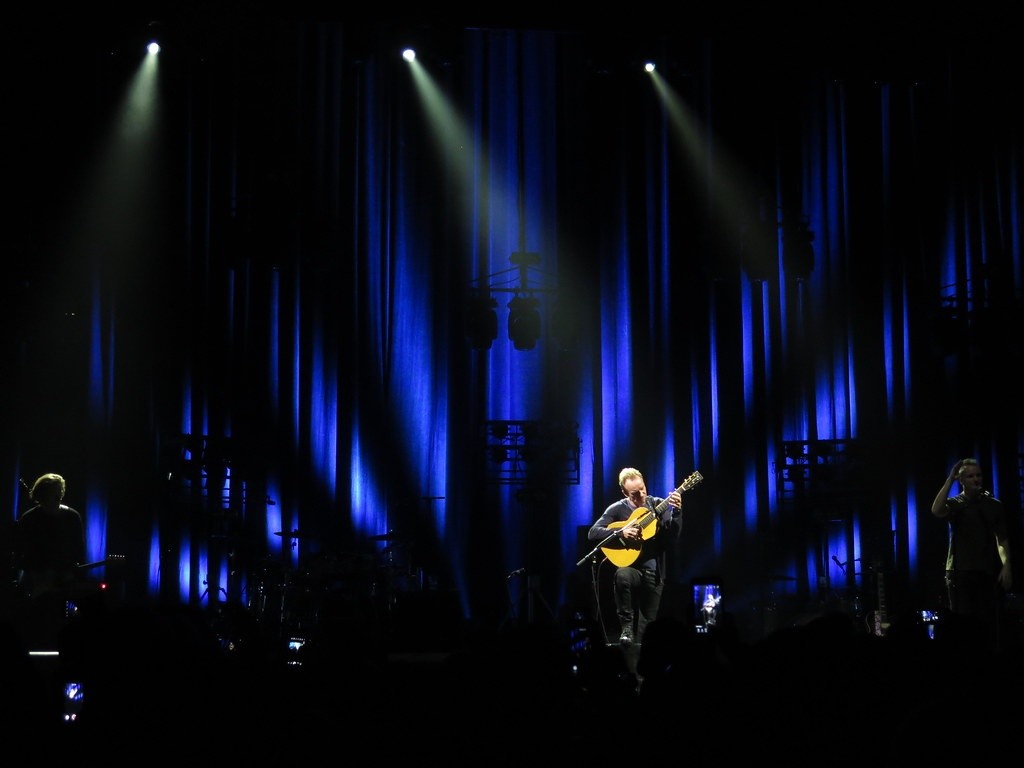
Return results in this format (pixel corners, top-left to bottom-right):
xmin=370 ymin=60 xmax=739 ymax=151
xmin=619 ymin=626 xmax=633 ymax=647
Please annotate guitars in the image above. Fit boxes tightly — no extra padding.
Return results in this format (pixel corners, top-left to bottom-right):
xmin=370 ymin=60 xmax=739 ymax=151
xmin=72 ymin=554 xmax=127 ymax=574
xmin=599 ymin=470 xmax=704 ymax=569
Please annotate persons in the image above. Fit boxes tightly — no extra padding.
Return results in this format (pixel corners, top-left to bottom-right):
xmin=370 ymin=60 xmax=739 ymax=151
xmin=588 ymin=466 xmax=683 ymax=648
xmin=11 ymin=473 xmax=85 ymax=606
xmin=931 ymin=458 xmax=1013 ymax=606
